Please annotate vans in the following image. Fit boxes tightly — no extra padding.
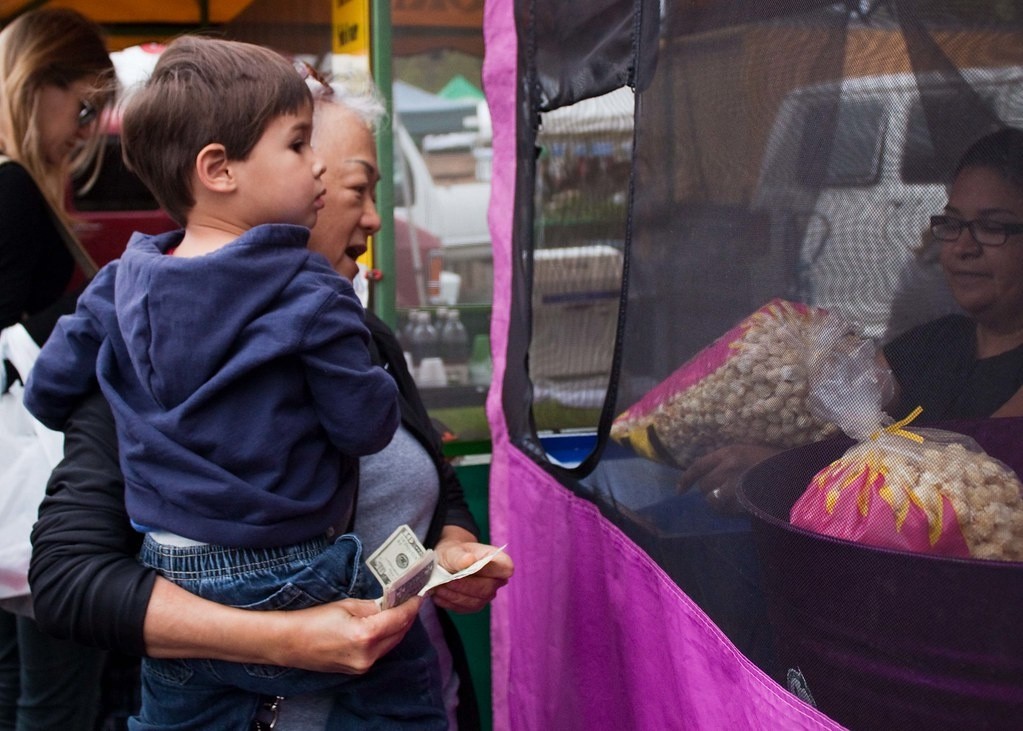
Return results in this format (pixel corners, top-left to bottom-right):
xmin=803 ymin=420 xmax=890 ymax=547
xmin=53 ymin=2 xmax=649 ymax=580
xmin=747 ymin=65 xmax=1023 ymax=340
xmin=55 ymin=102 xmax=440 ymax=341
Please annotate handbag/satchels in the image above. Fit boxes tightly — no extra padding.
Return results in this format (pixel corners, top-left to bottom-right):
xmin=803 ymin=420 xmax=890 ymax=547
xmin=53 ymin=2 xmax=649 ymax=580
xmin=0 ymin=323 xmax=64 ymax=616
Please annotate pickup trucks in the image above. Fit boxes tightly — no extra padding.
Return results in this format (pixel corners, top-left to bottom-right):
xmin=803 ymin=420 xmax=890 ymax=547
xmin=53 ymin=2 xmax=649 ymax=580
xmin=395 ymin=124 xmax=629 ymax=392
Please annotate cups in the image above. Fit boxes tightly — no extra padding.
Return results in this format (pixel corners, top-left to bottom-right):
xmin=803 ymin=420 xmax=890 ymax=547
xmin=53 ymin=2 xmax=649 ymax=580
xmin=419 ymin=358 xmax=447 ymax=388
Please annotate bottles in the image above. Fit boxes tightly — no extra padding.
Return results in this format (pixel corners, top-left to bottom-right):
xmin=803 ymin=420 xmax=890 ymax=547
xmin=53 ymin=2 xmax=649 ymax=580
xmin=394 ymin=306 xmax=470 ymax=389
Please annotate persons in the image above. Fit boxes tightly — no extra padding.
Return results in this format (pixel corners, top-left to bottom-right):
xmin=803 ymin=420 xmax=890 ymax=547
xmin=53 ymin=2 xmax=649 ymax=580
xmin=0 ymin=7 xmax=138 ymax=730
xmin=24 ymin=35 xmax=516 ymax=731
xmin=684 ymin=127 xmax=1022 ymax=524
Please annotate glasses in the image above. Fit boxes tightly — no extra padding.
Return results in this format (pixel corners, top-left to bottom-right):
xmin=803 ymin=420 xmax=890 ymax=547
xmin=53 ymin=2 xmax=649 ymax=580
xmin=930 ymin=215 xmax=1023 ymax=247
xmin=67 ymin=83 xmax=96 ymax=126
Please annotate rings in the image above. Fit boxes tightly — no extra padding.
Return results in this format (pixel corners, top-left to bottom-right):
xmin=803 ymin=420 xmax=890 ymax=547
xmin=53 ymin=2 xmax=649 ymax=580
xmin=713 ymin=489 xmax=728 ymax=509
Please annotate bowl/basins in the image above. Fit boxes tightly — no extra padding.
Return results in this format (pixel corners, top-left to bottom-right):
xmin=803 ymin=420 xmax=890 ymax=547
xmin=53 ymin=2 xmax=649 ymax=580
xmin=734 ymin=416 xmax=1023 ymax=662
